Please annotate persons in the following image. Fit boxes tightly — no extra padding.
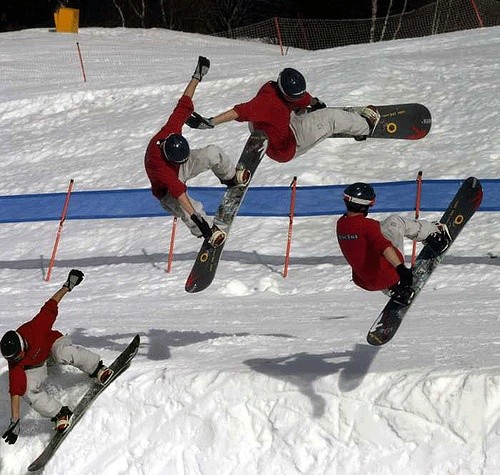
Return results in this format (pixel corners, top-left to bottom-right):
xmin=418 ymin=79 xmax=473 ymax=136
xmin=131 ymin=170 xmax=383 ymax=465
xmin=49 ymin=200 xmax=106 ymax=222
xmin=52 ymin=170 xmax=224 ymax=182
xmin=185 ymin=68 xmax=380 ymax=163
xmin=337 ymin=183 xmax=451 ymax=305
xmin=145 ymin=55 xmax=251 ymax=248
xmin=0 ymin=269 xmax=114 ymax=445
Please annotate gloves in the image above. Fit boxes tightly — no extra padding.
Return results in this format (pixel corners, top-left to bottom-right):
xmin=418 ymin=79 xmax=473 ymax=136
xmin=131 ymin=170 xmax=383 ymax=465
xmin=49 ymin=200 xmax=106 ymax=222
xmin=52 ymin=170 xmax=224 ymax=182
xmin=397 ymin=264 xmax=412 ymax=287
xmin=2 ymin=418 xmax=21 ymax=445
xmin=190 ymin=212 xmax=212 ymax=238
xmin=63 ymin=269 xmax=84 ymax=292
xmin=185 ymin=112 xmax=214 ymax=129
xmin=192 ymin=56 xmax=210 ymax=81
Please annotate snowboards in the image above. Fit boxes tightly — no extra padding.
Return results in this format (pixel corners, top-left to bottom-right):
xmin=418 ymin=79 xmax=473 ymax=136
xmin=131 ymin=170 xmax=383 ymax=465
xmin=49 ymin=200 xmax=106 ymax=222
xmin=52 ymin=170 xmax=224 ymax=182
xmin=185 ymin=130 xmax=269 ymax=293
xmin=296 ymin=102 xmax=431 ymax=141
xmin=367 ymin=176 xmax=483 ymax=346
xmin=28 ymin=332 xmax=140 ymax=473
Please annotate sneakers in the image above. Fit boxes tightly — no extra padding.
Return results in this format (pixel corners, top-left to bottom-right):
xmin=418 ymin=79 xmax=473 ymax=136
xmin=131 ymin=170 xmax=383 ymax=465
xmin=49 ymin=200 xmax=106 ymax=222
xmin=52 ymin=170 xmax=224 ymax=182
xmin=422 ymin=232 xmax=449 ymax=253
xmin=221 ymin=169 xmax=248 ymax=185
xmin=354 ymin=105 xmax=378 ymax=141
xmin=206 ymin=225 xmax=224 ymax=244
xmin=382 ymin=281 xmax=415 ymax=306
xmin=100 ymin=370 xmax=112 ymax=383
xmin=56 ymin=415 xmax=71 ymax=431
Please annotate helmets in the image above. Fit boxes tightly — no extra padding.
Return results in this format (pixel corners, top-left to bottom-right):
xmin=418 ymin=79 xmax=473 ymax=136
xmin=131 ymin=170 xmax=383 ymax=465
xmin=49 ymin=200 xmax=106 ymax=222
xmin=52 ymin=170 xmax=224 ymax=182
xmin=0 ymin=331 xmax=26 ymax=363
xmin=163 ymin=133 xmax=190 ymax=164
xmin=277 ymin=68 xmax=306 ymax=98
xmin=343 ymin=183 xmax=376 ymax=210
